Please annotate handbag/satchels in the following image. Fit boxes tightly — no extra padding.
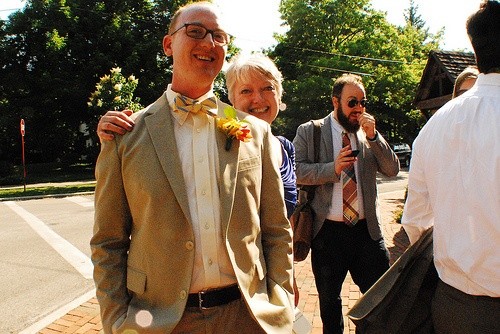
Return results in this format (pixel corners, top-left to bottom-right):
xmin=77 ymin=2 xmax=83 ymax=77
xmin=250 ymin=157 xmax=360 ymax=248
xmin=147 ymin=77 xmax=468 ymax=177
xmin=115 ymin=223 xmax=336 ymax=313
xmin=290 ymin=203 xmax=313 ymax=262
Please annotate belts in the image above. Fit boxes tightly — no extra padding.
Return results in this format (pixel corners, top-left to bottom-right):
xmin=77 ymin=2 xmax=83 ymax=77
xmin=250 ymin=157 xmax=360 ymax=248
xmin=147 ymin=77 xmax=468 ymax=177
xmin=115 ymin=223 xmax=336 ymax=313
xmin=186 ymin=284 xmax=240 ymax=310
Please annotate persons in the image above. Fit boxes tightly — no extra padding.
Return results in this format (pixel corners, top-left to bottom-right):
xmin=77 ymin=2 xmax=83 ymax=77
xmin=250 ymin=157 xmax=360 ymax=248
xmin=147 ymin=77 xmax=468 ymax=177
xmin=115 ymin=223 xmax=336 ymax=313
xmin=401 ymin=0 xmax=500 ymax=334
xmin=292 ymin=74 xmax=400 ymax=334
xmin=451 ymin=66 xmax=479 ymax=99
xmin=90 ymin=1 xmax=294 ymax=334
xmin=97 ymin=55 xmax=299 ymax=306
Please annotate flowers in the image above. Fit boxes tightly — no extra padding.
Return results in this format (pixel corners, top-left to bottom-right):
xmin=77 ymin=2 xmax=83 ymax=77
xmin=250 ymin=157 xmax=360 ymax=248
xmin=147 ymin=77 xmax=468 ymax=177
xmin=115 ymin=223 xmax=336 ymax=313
xmin=215 ymin=106 xmax=255 ymax=152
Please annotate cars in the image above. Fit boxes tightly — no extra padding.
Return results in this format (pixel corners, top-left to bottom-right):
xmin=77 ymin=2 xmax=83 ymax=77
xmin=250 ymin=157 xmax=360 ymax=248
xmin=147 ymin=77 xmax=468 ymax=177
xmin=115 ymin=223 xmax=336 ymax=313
xmin=389 ymin=142 xmax=411 ymax=167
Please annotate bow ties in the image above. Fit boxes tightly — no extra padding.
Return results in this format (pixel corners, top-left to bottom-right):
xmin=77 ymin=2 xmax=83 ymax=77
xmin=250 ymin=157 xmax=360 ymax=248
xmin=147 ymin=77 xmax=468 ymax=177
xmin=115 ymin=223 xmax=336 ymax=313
xmin=173 ymin=92 xmax=218 ymax=118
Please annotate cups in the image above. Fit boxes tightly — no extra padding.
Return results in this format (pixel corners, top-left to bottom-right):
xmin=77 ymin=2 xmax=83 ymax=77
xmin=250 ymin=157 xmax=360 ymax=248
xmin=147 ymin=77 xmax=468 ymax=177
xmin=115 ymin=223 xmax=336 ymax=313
xmin=347 ymin=140 xmax=360 ymax=158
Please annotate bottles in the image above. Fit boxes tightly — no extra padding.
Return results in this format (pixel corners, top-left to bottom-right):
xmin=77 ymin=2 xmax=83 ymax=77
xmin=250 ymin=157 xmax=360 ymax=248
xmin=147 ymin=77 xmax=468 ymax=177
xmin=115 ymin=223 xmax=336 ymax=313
xmin=115 ymin=310 xmax=153 ymax=334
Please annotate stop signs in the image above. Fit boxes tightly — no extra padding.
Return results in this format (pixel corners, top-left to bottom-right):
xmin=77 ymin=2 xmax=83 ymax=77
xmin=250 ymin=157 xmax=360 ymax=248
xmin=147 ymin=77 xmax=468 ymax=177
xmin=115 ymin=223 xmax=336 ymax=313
xmin=21 ymin=119 xmax=25 ymax=136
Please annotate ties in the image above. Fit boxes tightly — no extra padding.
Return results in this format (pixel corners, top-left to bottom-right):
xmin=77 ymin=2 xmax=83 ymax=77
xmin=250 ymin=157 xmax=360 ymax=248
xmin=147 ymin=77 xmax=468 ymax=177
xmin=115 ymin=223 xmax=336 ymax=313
xmin=341 ymin=130 xmax=360 ymax=227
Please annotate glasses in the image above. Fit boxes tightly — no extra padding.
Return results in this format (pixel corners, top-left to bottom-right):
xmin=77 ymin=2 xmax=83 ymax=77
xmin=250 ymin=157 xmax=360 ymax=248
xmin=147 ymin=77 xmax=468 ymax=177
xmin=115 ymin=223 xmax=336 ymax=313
xmin=169 ymin=24 xmax=233 ymax=47
xmin=455 ymin=90 xmax=467 ymax=96
xmin=335 ymin=95 xmax=369 ymax=109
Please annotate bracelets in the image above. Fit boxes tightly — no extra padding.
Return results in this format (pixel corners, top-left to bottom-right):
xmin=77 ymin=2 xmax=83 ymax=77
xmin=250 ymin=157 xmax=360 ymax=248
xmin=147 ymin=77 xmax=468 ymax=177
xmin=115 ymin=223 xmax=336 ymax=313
xmin=368 ymin=132 xmax=378 ymax=141
